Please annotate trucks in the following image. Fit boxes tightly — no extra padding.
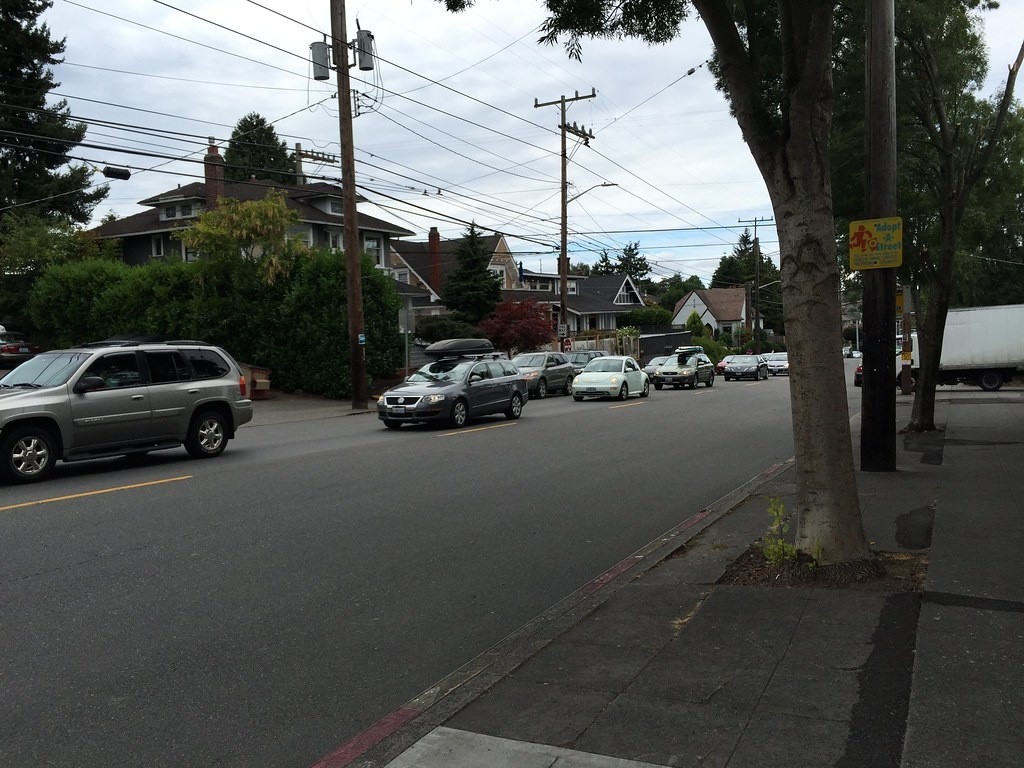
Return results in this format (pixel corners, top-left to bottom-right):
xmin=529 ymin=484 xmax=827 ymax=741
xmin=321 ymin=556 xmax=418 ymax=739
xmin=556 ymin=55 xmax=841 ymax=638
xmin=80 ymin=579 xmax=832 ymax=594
xmin=894 ymin=304 xmax=1024 ymax=395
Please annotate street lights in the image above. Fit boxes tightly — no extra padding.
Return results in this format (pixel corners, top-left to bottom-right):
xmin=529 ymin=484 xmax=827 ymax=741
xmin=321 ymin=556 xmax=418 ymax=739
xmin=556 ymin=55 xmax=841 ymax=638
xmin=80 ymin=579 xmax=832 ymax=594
xmin=566 ymin=178 xmax=619 ymax=204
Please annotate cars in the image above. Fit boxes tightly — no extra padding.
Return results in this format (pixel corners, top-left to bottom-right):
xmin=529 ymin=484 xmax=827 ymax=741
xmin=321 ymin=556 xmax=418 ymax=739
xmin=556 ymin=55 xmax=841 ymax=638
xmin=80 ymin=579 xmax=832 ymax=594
xmin=0 ymin=339 xmax=253 ymax=483
xmin=376 ymin=337 xmax=529 ymax=429
xmin=854 ymin=360 xmax=864 ymax=386
xmin=571 ymin=355 xmax=650 ymax=402
xmin=515 ymin=349 xmax=612 ymax=401
xmin=640 ymin=347 xmax=790 ymax=389
